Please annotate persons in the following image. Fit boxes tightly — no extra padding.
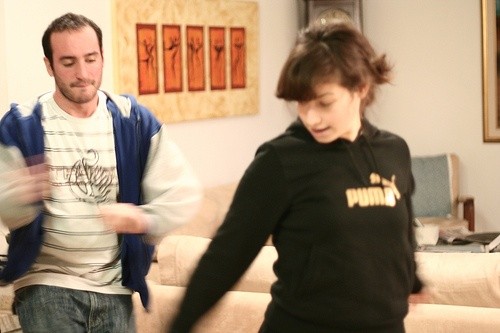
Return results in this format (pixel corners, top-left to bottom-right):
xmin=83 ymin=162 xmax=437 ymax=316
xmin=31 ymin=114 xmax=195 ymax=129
xmin=162 ymin=19 xmax=434 ymax=333
xmin=0 ymin=12 xmax=203 ymax=333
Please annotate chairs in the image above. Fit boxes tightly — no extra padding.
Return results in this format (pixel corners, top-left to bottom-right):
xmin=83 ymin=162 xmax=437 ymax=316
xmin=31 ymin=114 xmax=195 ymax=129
xmin=411 ymin=152 xmax=475 ymax=232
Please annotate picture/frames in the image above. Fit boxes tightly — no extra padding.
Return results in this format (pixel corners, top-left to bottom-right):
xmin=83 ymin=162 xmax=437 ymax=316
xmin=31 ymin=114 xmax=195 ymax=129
xmin=480 ymin=0 xmax=500 ymax=144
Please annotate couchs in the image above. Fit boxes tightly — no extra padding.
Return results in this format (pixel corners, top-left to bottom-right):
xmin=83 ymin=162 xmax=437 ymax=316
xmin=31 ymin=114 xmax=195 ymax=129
xmin=133 ymin=234 xmax=500 ymax=333
xmin=0 ymin=182 xmax=272 ymax=333
